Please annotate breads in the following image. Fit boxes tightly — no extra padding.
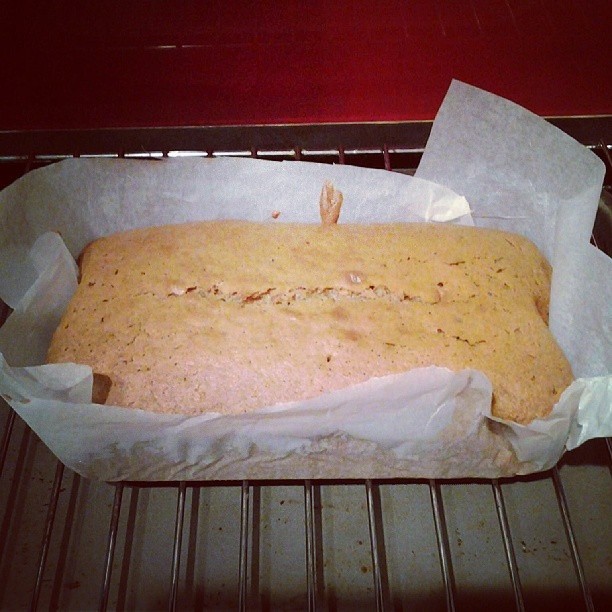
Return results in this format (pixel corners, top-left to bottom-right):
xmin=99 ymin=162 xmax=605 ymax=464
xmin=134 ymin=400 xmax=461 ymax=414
xmin=43 ymin=220 xmax=577 ymax=483
